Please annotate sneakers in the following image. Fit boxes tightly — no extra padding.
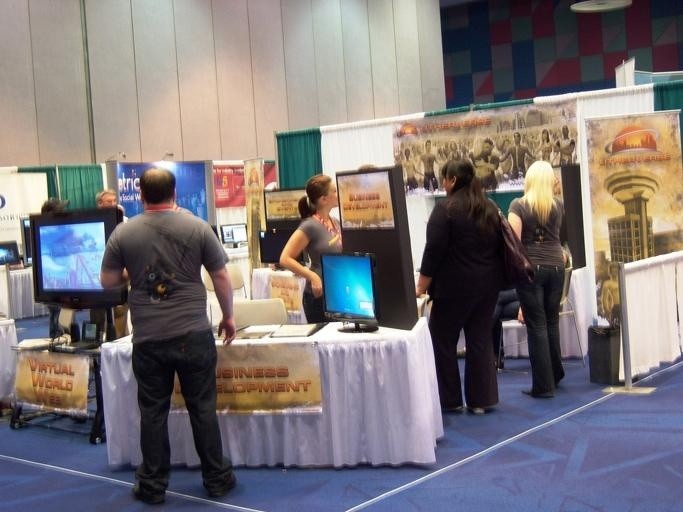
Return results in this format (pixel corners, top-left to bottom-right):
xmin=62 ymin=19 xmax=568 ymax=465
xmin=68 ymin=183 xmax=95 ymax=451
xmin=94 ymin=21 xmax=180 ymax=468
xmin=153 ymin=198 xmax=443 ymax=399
xmin=204 ymin=471 xmax=238 ymax=497
xmin=133 ymin=479 xmax=169 ymax=504
xmin=463 ymin=400 xmax=486 ymax=415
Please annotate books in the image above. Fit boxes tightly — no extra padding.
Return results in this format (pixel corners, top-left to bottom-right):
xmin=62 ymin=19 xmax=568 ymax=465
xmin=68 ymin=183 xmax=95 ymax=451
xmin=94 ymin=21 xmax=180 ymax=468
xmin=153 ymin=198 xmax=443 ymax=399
xmin=272 ymin=324 xmax=316 ymax=336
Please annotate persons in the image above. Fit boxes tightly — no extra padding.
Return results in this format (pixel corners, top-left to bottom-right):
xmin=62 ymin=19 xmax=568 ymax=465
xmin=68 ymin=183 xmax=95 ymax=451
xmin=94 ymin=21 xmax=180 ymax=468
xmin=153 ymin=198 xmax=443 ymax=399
xmin=100 ymin=167 xmax=237 ymax=504
xmin=90 ymin=189 xmax=132 ymax=338
xmin=493 ymin=210 xmax=570 ymax=373
xmin=41 ymin=196 xmax=71 ymax=340
xmin=508 ymin=160 xmax=566 ymax=399
xmin=601 ymin=262 xmax=620 ymax=327
xmin=278 ymin=174 xmax=342 ymax=324
xmin=394 ymin=125 xmax=576 ymax=191
xmin=414 ymin=159 xmax=506 ymax=415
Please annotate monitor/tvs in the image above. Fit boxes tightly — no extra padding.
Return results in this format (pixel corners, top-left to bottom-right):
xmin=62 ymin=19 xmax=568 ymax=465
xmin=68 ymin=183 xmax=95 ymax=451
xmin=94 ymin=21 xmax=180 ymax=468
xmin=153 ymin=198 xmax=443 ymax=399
xmin=221 ymin=224 xmax=248 ymax=248
xmin=259 ymin=229 xmax=304 ymax=263
xmin=320 ymin=252 xmax=380 ymax=333
xmin=0 ymin=217 xmax=32 ymax=267
xmin=30 ymin=208 xmax=128 ymax=309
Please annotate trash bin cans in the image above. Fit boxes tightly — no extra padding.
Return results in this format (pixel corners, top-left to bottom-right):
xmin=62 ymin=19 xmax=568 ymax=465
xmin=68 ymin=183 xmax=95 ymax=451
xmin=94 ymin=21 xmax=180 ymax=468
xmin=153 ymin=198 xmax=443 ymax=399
xmin=588 ymin=325 xmax=621 ymax=386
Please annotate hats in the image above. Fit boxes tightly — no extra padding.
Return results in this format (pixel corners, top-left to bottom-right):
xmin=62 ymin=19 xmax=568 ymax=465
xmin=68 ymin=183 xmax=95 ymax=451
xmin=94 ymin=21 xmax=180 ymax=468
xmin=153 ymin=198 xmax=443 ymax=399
xmin=42 ymin=196 xmax=71 ymax=212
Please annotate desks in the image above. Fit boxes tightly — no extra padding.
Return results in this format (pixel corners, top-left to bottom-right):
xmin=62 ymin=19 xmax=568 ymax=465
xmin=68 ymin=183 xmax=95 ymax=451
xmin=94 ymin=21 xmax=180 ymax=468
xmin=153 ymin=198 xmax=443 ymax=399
xmin=99 ymin=316 xmax=446 ymax=468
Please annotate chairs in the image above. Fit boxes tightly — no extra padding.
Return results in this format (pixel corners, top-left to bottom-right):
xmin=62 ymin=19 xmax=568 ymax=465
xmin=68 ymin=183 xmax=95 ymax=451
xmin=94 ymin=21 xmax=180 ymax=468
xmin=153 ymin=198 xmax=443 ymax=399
xmin=232 ymin=298 xmax=288 ymax=329
xmin=202 ymin=264 xmax=249 ymax=328
